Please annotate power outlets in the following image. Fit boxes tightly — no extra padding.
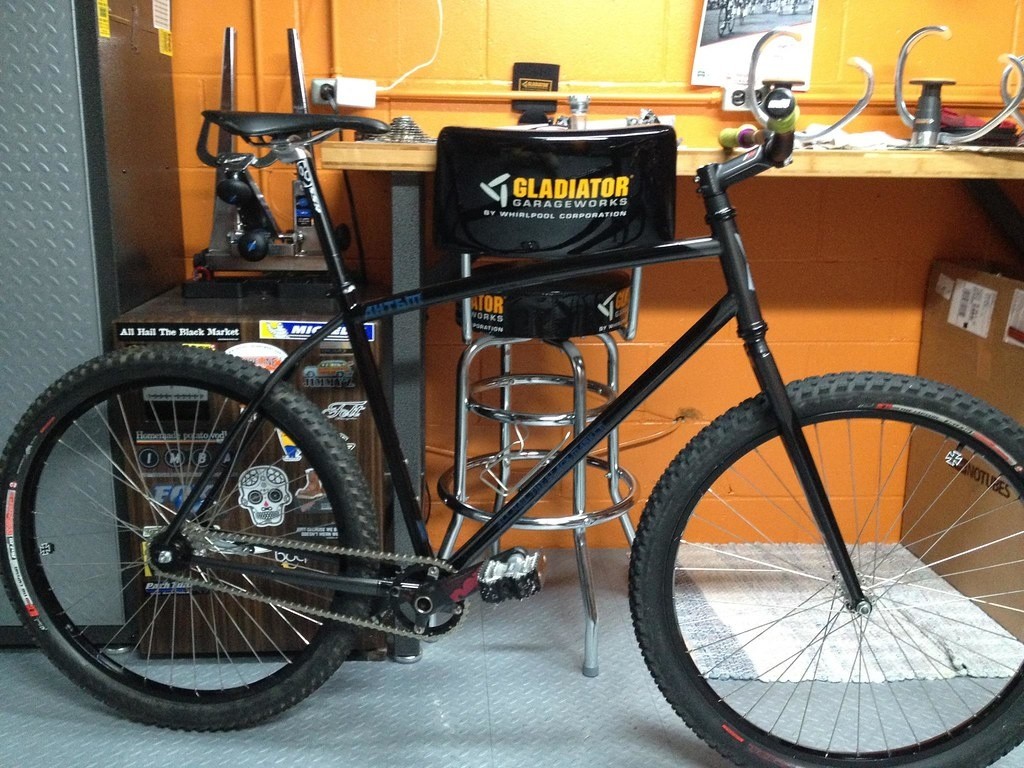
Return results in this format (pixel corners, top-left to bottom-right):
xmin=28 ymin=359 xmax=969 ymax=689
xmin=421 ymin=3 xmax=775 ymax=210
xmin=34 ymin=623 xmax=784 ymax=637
xmin=311 ymin=79 xmax=336 ymax=107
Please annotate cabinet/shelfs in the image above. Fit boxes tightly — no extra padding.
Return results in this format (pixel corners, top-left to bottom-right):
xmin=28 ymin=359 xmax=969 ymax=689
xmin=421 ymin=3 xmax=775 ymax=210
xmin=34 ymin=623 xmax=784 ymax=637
xmin=115 ymin=280 xmax=393 ymax=659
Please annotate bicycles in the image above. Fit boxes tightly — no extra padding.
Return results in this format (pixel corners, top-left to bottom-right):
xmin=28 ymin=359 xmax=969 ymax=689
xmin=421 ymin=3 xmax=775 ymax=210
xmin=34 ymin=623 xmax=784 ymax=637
xmin=716 ymin=2 xmax=739 ymax=39
xmin=1 ymin=28 xmax=1023 ymax=768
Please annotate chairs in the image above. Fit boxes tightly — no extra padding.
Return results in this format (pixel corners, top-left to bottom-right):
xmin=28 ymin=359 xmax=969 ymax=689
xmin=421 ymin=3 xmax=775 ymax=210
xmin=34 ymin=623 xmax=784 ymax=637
xmin=425 ymin=124 xmax=681 ymax=680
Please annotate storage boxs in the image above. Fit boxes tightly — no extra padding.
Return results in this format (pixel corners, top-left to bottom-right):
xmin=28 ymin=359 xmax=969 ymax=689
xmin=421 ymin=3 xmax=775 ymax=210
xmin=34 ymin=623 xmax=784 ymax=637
xmin=898 ymin=254 xmax=1023 ymax=648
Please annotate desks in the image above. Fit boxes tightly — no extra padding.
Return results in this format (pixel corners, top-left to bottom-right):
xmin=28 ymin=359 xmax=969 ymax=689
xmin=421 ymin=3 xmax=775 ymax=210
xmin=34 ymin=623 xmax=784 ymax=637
xmin=318 ymin=135 xmax=1024 ymax=666
